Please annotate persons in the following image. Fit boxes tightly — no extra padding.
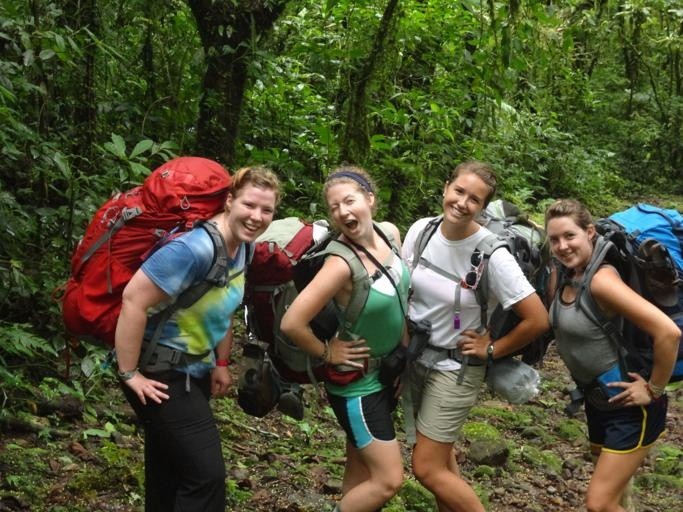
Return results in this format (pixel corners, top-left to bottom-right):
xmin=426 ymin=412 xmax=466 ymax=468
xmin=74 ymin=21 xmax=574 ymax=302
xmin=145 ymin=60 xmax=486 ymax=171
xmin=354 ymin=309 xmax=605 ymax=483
xmin=279 ymin=165 xmax=411 ymax=512
xmin=544 ymin=200 xmax=682 ymax=512
xmin=113 ymin=165 xmax=279 ymax=512
xmin=403 ymin=160 xmax=552 ymax=512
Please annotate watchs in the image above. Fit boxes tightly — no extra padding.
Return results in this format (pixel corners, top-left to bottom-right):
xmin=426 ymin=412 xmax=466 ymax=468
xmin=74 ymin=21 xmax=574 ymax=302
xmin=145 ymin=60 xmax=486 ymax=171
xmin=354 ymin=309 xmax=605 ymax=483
xmin=487 ymin=340 xmax=495 ymax=363
xmin=118 ymin=368 xmax=137 ymax=380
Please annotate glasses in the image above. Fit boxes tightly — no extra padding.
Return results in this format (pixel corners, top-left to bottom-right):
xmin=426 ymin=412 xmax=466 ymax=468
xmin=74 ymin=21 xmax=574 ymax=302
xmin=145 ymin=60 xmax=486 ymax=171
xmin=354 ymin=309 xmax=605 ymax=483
xmin=465 ymin=250 xmax=484 ymax=291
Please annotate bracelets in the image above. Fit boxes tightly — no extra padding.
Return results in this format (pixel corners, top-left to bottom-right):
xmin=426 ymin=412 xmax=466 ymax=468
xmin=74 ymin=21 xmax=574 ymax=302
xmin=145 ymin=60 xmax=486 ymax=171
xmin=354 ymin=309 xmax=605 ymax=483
xmin=215 ymin=357 xmax=229 ymax=368
xmin=317 ymin=343 xmax=329 ymax=361
xmin=646 ymin=380 xmax=666 ymax=401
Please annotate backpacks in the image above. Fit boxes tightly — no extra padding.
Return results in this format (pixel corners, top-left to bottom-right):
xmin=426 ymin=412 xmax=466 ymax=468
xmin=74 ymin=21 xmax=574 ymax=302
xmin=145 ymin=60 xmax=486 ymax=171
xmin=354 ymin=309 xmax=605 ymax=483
xmin=243 ymin=216 xmax=402 ymax=384
xmin=553 ymin=202 xmax=683 ymax=418
xmin=62 ymin=157 xmax=251 ymax=351
xmin=409 ymin=199 xmax=554 ymax=366
